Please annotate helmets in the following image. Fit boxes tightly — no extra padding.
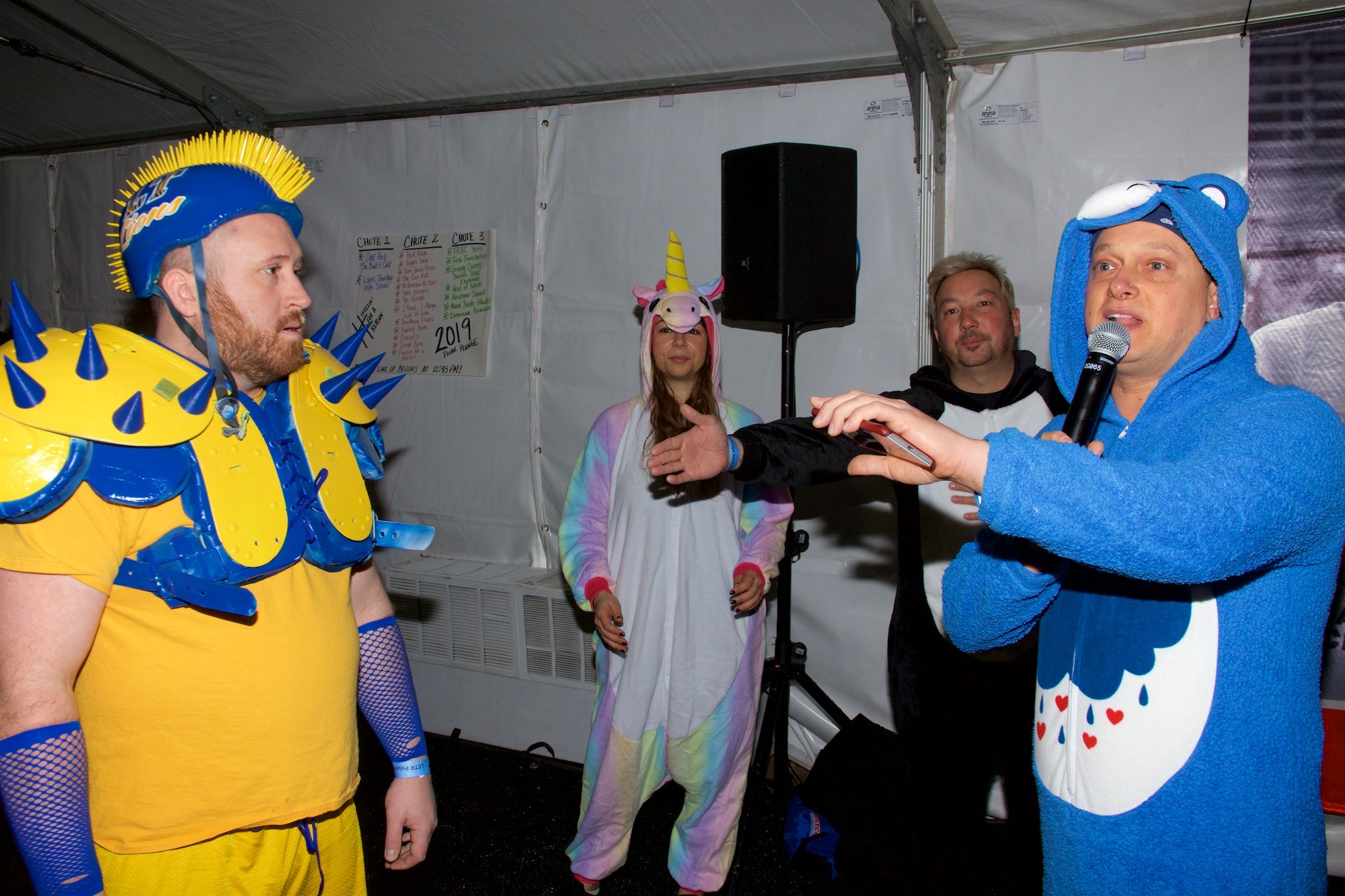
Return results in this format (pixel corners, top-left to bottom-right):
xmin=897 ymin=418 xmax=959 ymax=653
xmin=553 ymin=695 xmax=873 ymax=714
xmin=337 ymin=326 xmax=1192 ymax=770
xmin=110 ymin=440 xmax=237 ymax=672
xmin=103 ymin=126 xmax=316 ymax=299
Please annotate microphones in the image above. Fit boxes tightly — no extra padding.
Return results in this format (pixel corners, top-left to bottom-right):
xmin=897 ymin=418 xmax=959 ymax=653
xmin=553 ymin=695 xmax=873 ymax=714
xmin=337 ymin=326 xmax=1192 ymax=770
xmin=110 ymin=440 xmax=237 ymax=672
xmin=1061 ymin=322 xmax=1130 ymax=447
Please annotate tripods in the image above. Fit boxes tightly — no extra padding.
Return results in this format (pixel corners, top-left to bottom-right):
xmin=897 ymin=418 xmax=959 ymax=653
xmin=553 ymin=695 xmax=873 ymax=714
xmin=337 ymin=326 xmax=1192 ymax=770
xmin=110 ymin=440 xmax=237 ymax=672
xmin=746 ymin=323 xmax=855 ymax=873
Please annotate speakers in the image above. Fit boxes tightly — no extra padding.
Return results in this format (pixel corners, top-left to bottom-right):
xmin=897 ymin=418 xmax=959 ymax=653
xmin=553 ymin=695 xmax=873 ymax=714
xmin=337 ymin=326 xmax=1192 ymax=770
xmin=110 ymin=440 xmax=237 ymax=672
xmin=720 ymin=142 xmax=856 ymax=322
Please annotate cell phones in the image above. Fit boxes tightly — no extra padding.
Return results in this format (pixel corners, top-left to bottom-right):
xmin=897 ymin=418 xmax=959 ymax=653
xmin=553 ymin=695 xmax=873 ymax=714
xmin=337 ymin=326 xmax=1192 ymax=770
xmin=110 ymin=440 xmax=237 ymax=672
xmin=811 ymin=407 xmax=935 ymax=471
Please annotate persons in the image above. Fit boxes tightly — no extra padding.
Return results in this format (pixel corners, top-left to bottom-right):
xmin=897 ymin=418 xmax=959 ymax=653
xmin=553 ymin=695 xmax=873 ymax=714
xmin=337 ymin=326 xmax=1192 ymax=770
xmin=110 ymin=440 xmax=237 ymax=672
xmin=809 ymin=173 xmax=1345 ymax=896
xmin=558 ymin=231 xmax=795 ymax=896
xmin=646 ymin=253 xmax=1069 ymax=896
xmin=0 ymin=129 xmax=437 ymax=896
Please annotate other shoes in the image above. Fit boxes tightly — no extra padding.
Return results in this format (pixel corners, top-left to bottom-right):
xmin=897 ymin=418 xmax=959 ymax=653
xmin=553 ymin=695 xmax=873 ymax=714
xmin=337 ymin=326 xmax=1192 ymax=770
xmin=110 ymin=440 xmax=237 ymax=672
xmin=541 ymin=877 xmax=600 ymax=896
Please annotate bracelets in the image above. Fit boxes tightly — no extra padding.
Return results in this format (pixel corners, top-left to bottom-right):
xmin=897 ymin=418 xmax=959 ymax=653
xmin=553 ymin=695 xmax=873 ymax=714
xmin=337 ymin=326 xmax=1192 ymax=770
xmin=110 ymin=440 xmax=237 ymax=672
xmin=722 ymin=436 xmax=740 ymax=472
xmin=392 ymin=757 xmax=430 ymax=778
